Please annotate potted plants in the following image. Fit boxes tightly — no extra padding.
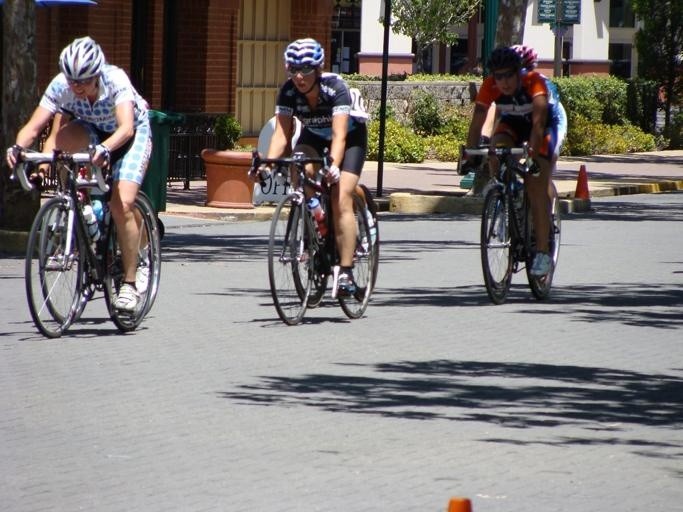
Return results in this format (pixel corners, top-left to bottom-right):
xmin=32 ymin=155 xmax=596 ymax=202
xmin=200 ymin=117 xmax=259 ymax=208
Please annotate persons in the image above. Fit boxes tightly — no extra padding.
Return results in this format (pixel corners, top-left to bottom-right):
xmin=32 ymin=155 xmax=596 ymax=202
xmin=248 ymin=38 xmax=371 ymax=295
xmin=467 ymin=44 xmax=568 ymax=275
xmin=30 ymin=66 xmax=151 ymax=295
xmin=7 ymin=36 xmax=153 ymax=312
xmin=509 ymin=45 xmax=567 ymax=174
xmin=352 ymin=185 xmax=377 ymax=256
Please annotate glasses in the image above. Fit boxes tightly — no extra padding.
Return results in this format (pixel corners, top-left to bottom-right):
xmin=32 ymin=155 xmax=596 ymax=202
xmin=64 ymin=75 xmax=93 ymax=85
xmin=287 ymin=65 xmax=316 ymax=75
xmin=494 ymin=70 xmax=514 ymax=79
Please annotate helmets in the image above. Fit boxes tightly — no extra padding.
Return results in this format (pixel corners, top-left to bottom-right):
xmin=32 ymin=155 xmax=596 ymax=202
xmin=284 ymin=38 xmax=325 ymax=67
xmin=488 ymin=46 xmax=521 ymax=73
xmin=510 ymin=44 xmax=539 ymax=71
xmin=59 ymin=36 xmax=105 ymax=80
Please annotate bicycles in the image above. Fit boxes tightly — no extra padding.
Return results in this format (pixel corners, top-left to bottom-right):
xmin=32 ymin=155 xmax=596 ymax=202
xmin=456 ymin=143 xmax=553 ymax=306
xmin=257 ymin=154 xmax=381 ymax=309
xmin=246 ymin=149 xmax=376 ymax=328
xmin=475 ymin=143 xmax=560 ymax=292
xmin=8 ymin=143 xmax=152 ymax=339
xmin=28 ymin=146 xmax=160 ymax=326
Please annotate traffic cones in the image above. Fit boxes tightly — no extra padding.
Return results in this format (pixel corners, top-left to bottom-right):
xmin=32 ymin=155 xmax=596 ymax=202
xmin=574 ymin=164 xmax=590 ymax=200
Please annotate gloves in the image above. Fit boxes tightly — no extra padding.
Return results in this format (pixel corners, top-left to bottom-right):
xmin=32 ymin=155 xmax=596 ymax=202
xmin=7 ymin=144 xmax=20 ymax=157
xmin=95 ymin=144 xmax=112 ymax=164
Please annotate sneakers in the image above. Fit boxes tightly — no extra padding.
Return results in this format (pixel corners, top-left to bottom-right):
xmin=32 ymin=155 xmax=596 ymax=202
xmin=114 ymin=283 xmax=140 ymax=311
xmin=337 ymin=267 xmax=356 ymax=294
xmin=530 ymin=252 xmax=552 ymax=276
